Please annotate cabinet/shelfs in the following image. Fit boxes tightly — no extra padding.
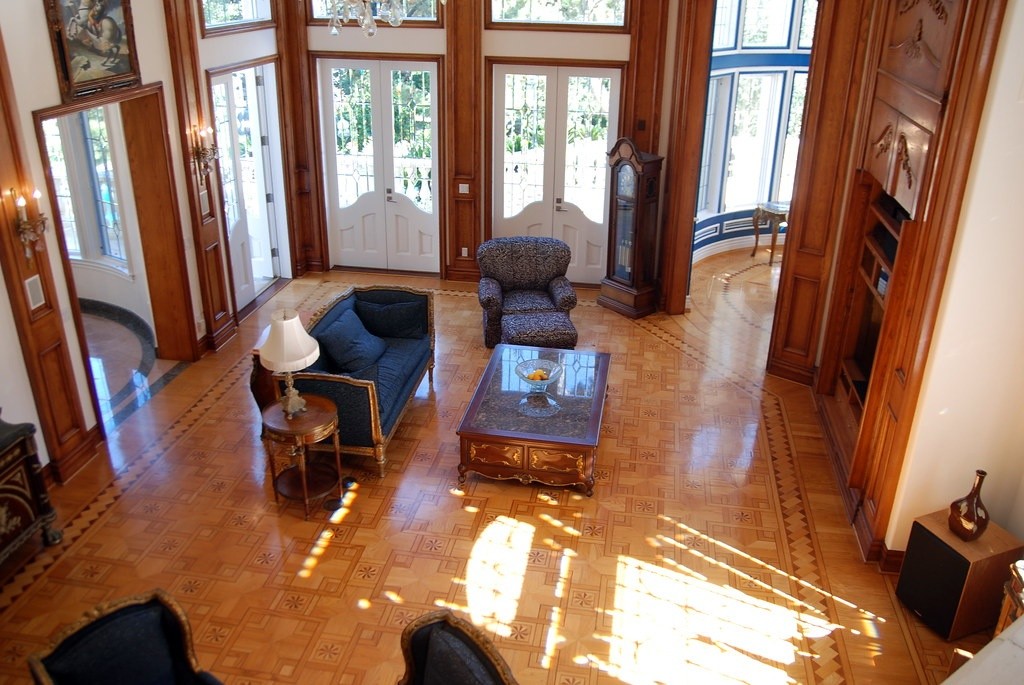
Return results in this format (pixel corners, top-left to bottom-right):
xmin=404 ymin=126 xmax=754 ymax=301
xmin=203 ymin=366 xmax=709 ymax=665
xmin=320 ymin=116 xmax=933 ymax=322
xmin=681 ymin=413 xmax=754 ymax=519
xmin=833 ymin=180 xmax=902 ymax=453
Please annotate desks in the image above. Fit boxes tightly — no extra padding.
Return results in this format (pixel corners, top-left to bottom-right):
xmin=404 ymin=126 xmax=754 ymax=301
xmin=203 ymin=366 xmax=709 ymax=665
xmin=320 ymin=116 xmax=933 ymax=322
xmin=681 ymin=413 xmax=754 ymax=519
xmin=751 ymin=199 xmax=792 ymax=266
xmin=0 ymin=407 xmax=65 ymax=565
xmin=894 ymin=509 xmax=1024 ymax=644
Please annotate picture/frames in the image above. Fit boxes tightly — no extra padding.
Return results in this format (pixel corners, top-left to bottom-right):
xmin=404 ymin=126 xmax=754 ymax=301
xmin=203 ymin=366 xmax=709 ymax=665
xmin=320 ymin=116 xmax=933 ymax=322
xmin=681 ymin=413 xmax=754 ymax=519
xmin=43 ymin=0 xmax=143 ymax=105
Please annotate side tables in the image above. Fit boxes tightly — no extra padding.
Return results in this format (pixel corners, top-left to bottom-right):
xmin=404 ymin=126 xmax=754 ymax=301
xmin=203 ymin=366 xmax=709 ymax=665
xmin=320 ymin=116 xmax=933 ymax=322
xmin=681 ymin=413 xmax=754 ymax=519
xmin=259 ymin=394 xmax=344 ymax=520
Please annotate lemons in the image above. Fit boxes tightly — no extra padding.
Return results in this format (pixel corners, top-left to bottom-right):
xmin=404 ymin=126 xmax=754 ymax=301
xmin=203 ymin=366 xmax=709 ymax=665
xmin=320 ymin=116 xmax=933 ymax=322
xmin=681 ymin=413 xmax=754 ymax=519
xmin=528 ymin=369 xmax=548 ymax=381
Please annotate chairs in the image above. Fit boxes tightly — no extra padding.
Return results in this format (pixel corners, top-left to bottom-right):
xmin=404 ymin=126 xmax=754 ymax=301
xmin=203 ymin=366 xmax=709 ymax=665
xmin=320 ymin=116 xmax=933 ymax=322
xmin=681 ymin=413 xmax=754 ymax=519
xmin=396 ymin=607 xmax=519 ymax=685
xmin=25 ymin=587 xmax=225 ymax=685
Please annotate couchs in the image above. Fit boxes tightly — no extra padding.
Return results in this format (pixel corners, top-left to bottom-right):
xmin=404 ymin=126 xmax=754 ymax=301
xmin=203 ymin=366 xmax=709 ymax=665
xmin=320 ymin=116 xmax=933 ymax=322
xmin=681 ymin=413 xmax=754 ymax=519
xmin=476 ymin=237 xmax=578 ymax=350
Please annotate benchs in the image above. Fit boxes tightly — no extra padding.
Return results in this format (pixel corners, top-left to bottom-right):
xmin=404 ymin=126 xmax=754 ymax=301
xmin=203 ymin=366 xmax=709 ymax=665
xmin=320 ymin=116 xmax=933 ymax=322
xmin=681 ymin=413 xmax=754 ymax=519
xmin=270 ymin=283 xmax=436 ymax=478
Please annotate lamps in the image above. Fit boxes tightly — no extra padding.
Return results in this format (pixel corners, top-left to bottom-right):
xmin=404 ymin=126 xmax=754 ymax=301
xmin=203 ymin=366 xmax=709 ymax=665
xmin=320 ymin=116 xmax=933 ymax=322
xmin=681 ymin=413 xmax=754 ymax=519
xmin=10 ymin=187 xmax=48 ymax=271
xmin=187 ymin=124 xmax=225 ymax=185
xmin=259 ymin=307 xmax=321 ymax=420
xmin=327 ymin=0 xmax=448 ymax=40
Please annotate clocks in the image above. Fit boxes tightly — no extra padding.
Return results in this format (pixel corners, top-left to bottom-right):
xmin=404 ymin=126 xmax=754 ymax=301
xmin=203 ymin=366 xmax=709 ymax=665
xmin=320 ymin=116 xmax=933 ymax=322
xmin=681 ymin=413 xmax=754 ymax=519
xmin=595 ymin=136 xmax=666 ymax=319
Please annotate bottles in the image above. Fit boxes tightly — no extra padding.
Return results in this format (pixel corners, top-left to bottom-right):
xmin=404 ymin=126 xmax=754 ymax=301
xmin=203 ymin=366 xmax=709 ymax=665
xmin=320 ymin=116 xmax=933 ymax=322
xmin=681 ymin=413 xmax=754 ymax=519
xmin=948 ymin=470 xmax=990 ymax=543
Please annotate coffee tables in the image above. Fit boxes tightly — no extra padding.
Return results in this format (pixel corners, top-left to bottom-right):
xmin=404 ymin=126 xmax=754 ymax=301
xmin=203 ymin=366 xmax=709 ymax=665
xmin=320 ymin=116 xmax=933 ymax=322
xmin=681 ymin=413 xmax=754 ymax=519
xmin=454 ymin=344 xmax=612 ymax=499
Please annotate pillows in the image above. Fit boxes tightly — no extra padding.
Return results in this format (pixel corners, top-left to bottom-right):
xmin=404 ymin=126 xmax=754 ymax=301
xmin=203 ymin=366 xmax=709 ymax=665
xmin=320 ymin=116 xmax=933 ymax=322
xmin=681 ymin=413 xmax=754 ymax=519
xmin=319 ymin=363 xmax=385 ymax=414
xmin=315 ymin=308 xmax=388 ymax=372
xmin=354 ymin=300 xmax=425 ymax=340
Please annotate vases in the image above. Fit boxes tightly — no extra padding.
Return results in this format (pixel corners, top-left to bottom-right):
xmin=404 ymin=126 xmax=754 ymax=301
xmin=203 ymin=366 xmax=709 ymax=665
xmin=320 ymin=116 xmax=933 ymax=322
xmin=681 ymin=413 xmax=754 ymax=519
xmin=948 ymin=469 xmax=990 ymax=542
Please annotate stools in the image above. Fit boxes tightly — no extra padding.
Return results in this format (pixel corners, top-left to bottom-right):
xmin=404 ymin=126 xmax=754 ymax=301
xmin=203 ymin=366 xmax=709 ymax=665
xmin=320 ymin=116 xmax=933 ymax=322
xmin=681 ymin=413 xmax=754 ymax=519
xmin=499 ymin=312 xmax=577 ymax=350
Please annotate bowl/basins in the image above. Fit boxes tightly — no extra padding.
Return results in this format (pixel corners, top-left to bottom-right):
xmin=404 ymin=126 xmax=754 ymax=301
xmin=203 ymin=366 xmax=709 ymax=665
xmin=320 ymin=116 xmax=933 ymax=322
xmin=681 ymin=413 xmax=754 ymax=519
xmin=515 ymin=359 xmax=563 ymax=388
xmin=517 ymin=392 xmax=561 ymax=418
